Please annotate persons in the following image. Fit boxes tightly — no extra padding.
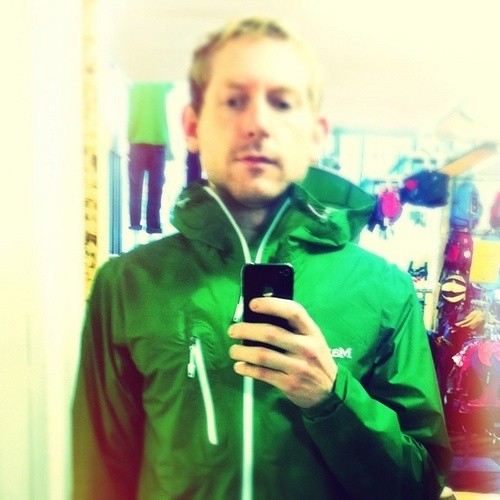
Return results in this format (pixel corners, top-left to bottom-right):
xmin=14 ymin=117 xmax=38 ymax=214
xmin=110 ymin=31 xmax=183 ymax=232
xmin=64 ymin=14 xmax=455 ymax=499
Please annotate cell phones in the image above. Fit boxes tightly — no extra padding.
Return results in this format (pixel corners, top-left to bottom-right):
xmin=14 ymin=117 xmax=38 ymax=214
xmin=241 ymin=262 xmax=294 ymax=354
